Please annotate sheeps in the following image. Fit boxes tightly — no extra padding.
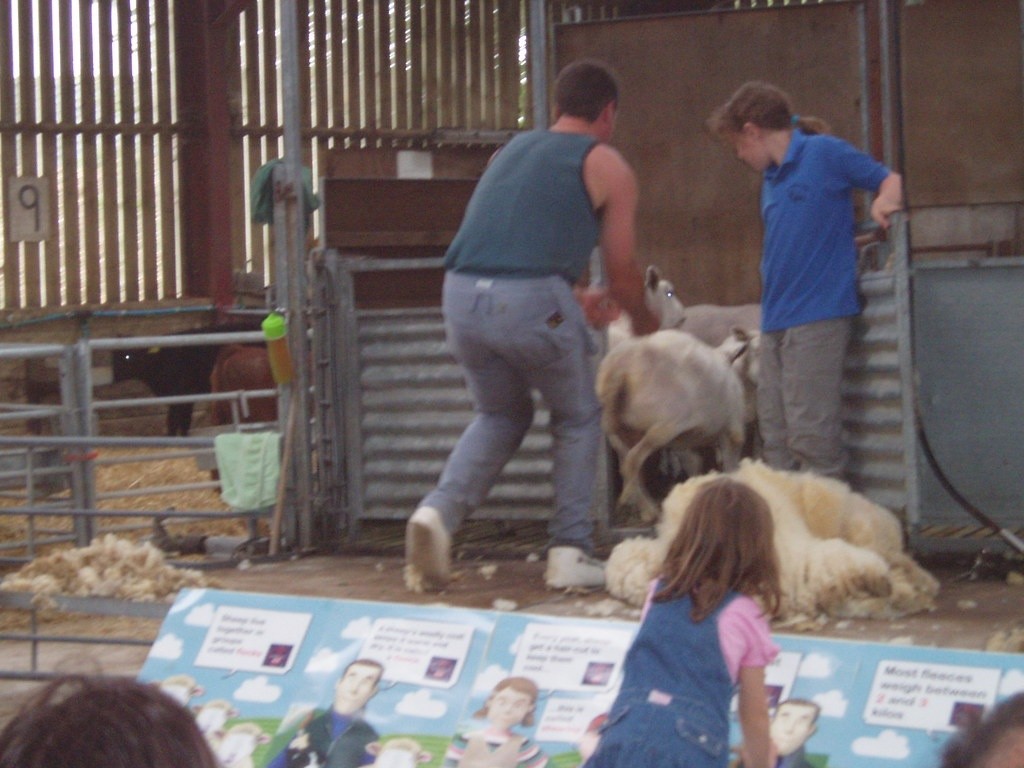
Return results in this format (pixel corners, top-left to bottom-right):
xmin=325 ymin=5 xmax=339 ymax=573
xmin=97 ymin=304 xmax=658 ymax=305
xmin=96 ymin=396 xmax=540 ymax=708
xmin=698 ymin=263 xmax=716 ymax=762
xmin=112 ymin=320 xmax=315 ymax=438
xmin=591 ymin=264 xmax=763 ymax=525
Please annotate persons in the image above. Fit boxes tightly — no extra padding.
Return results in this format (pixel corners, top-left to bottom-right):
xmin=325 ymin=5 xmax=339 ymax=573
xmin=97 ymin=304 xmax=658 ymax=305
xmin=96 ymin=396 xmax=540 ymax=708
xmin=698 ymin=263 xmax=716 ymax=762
xmin=707 ymin=82 xmax=903 ymax=485
xmin=580 ymin=479 xmax=783 ymax=768
xmin=407 ymin=60 xmax=663 ymax=594
xmin=0 ymin=674 xmax=215 ymax=768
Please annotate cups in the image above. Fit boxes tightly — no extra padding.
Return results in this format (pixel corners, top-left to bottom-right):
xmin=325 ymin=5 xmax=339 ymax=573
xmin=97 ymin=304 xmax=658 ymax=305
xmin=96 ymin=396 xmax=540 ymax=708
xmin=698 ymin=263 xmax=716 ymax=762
xmin=261 ymin=312 xmax=294 ymax=385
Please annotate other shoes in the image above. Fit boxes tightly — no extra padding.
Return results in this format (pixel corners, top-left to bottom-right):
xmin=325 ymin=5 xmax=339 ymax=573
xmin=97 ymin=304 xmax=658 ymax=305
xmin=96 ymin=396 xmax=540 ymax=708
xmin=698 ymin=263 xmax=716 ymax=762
xmin=544 ymin=545 xmax=605 ymax=591
xmin=405 ymin=507 xmax=453 ymax=592
